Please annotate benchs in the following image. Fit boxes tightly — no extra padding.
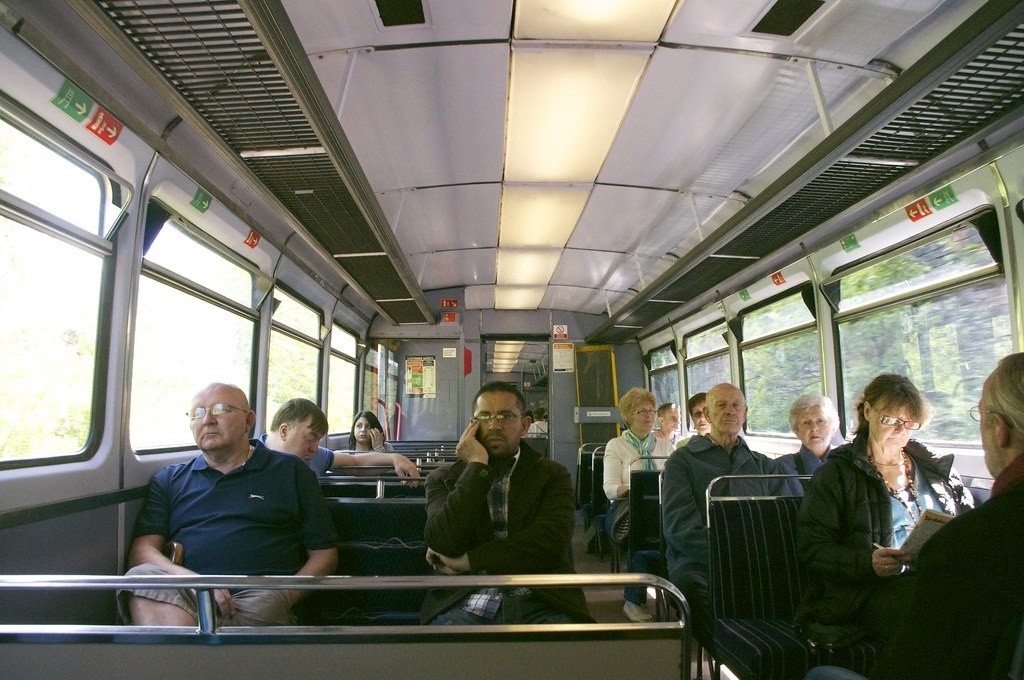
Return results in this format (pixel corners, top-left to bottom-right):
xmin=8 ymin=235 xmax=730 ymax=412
xmin=0 ymin=440 xmax=885 ymax=680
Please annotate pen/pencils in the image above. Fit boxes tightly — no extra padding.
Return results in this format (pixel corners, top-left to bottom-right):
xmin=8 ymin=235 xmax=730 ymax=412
xmin=873 ymin=543 xmax=906 ymax=565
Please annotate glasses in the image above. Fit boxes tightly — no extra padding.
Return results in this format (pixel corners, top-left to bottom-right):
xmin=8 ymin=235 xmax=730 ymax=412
xmin=969 ymin=406 xmax=1014 ymax=428
xmin=692 ymin=412 xmax=702 ymax=417
xmin=634 ymin=408 xmax=658 ymax=415
xmin=470 ymin=412 xmax=524 ymax=423
xmin=185 ymin=403 xmax=247 ymax=419
xmin=873 ymin=410 xmax=921 ymax=430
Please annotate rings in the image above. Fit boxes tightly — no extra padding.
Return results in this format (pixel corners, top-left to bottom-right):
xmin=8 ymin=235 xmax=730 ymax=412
xmin=885 ymin=565 xmax=888 ymax=571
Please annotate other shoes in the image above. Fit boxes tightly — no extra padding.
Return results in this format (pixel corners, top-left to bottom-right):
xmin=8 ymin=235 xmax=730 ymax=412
xmin=622 ymin=603 xmax=654 ymax=623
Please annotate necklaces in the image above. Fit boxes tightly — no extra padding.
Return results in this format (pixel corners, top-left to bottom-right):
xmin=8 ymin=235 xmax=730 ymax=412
xmin=866 ymin=448 xmax=923 ymax=524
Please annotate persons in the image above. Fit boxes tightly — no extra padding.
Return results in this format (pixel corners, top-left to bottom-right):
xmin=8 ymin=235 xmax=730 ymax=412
xmin=259 ymin=398 xmax=420 ymax=487
xmin=602 ymin=385 xmax=711 ymax=622
xmin=422 ymin=379 xmax=598 ymax=626
xmin=116 ymin=382 xmax=340 ymax=626
xmin=662 ymin=384 xmax=839 ymax=645
xmin=348 ymin=411 xmax=394 ymax=452
xmin=653 ymin=403 xmax=686 ymax=449
xmin=804 ymin=352 xmax=1024 ymax=680
xmin=521 ymin=407 xmax=548 ymax=438
xmin=796 ymin=374 xmax=979 ymax=637
